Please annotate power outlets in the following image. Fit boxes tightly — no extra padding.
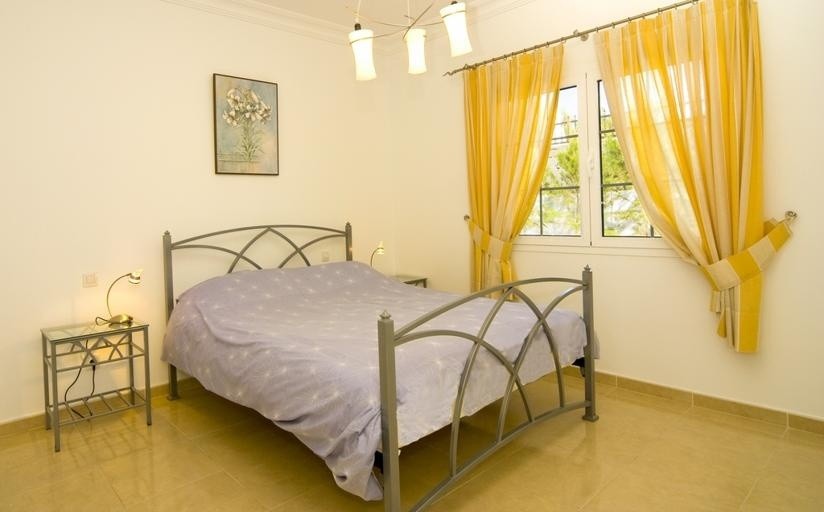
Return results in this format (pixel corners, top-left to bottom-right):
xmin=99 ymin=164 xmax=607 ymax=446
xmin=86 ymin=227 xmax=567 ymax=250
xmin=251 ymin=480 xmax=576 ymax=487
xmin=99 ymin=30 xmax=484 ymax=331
xmin=87 ymin=358 xmax=97 ymax=369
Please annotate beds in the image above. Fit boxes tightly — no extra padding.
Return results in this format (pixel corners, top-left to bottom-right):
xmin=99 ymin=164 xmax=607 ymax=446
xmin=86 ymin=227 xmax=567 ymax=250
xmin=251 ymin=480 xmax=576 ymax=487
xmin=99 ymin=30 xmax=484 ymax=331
xmin=161 ymin=222 xmax=599 ymax=511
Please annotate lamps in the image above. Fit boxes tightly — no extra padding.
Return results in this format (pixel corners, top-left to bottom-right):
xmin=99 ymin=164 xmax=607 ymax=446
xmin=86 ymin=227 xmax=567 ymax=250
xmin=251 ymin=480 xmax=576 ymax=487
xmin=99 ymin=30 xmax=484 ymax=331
xmin=106 ymin=271 xmax=141 ymax=324
xmin=370 ymin=246 xmax=384 ymax=268
xmin=348 ymin=1 xmax=473 ymax=82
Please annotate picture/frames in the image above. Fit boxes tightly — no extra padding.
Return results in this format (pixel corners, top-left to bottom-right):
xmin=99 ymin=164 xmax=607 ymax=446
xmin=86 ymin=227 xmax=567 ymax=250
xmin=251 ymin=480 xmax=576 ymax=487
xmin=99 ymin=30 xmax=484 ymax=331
xmin=213 ymin=73 xmax=279 ymax=176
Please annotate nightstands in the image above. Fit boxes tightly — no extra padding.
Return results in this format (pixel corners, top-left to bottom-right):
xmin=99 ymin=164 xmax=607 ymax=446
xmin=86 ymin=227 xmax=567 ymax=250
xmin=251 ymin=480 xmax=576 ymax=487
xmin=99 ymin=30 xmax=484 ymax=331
xmin=40 ymin=314 xmax=153 ymax=452
xmin=394 ymin=274 xmax=428 ymax=288
